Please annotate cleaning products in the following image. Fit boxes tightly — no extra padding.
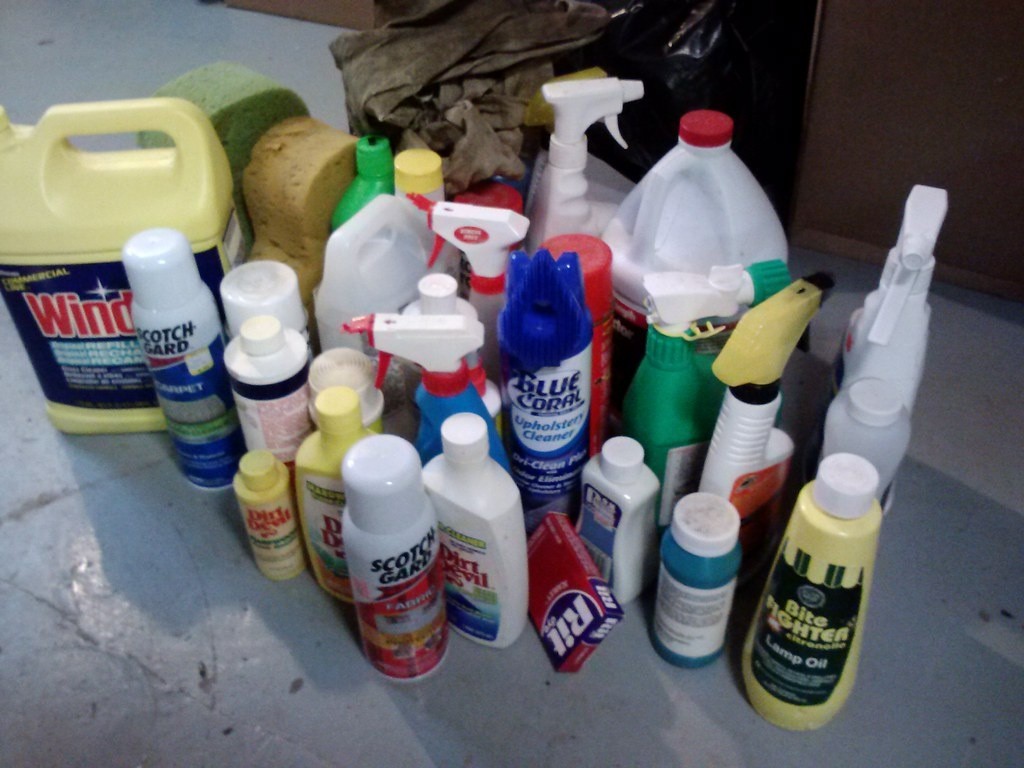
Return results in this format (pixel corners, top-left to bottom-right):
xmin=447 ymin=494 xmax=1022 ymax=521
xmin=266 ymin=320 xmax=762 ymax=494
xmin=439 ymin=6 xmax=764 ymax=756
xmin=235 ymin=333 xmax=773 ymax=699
xmin=0 ymin=56 xmax=960 ymax=736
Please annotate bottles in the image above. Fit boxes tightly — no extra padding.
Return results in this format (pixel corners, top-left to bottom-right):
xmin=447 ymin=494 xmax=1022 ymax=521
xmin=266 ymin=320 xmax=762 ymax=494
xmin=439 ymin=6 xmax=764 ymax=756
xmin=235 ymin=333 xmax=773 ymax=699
xmin=595 ymin=109 xmax=790 ymax=435
